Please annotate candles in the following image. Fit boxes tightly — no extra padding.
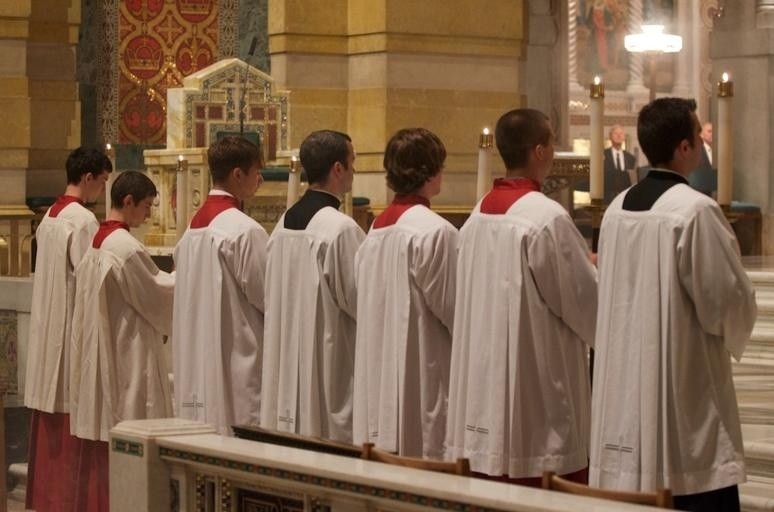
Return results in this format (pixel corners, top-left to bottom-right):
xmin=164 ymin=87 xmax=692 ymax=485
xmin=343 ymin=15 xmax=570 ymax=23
xmin=286 ymin=155 xmax=301 ymax=211
xmin=714 ymin=73 xmax=730 ymax=205
xmin=589 ymin=76 xmax=604 ymax=200
xmin=175 ymin=154 xmax=188 ymax=243
xmin=476 ymin=128 xmax=494 ymax=204
xmin=104 ymin=143 xmax=116 ymax=225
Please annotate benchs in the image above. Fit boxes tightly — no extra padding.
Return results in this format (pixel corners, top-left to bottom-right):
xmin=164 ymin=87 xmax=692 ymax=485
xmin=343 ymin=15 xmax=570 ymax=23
xmin=232 ymin=425 xmax=362 ymax=458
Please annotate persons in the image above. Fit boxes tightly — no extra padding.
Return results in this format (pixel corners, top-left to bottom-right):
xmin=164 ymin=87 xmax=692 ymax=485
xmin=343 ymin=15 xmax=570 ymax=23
xmin=578 ymin=97 xmax=759 ymax=512
xmin=66 ymin=169 xmax=175 ymax=512
xmin=597 ymin=123 xmax=637 ymax=201
xmin=161 ymin=133 xmax=272 ymax=441
xmin=349 ymin=126 xmax=464 ymax=463
xmin=684 ymin=121 xmax=717 ymax=204
xmin=257 ymin=128 xmax=370 ymax=454
xmin=20 ymin=145 xmax=113 ymax=512
xmin=443 ymin=106 xmax=607 ymax=484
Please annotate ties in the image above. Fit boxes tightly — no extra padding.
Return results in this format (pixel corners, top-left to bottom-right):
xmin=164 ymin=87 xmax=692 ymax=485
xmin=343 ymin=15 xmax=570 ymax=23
xmin=615 ymin=152 xmax=621 ymax=173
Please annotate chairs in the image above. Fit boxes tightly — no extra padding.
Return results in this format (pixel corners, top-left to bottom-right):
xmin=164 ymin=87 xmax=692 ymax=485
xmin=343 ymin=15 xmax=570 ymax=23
xmin=543 ymin=471 xmax=674 ymax=507
xmin=362 ymin=443 xmax=470 ymax=477
